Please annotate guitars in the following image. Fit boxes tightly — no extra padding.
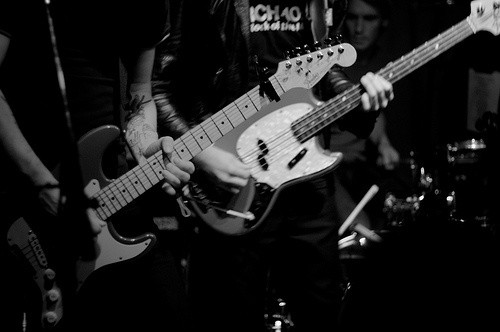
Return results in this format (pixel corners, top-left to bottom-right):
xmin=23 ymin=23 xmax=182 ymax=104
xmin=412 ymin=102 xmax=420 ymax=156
xmin=7 ymin=33 xmax=358 ymax=331
xmin=184 ymin=0 xmax=500 ymax=239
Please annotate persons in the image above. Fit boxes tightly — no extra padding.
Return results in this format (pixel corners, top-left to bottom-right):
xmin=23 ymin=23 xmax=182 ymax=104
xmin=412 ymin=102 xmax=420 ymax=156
xmin=151 ymin=0 xmax=395 ymax=332
xmin=0 ymin=0 xmax=196 ymax=332
xmin=314 ymin=0 xmax=400 ymax=249
